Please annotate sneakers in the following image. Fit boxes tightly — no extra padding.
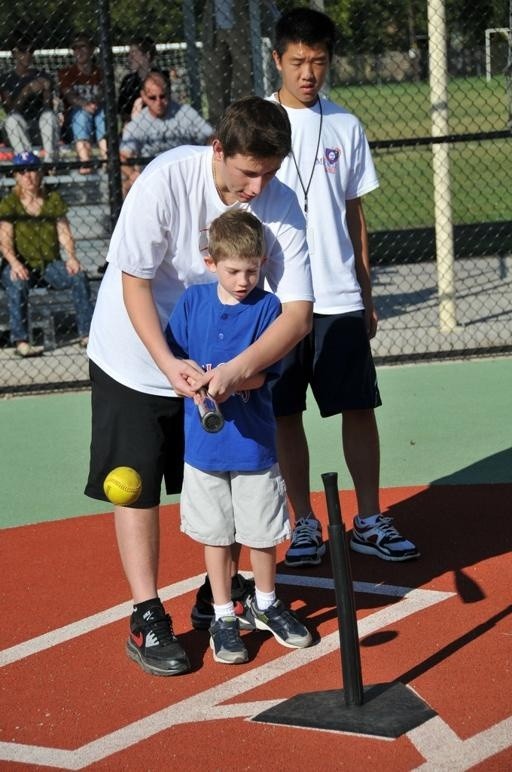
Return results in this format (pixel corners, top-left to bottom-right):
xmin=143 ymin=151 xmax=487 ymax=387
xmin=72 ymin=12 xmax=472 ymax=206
xmin=126 ymin=607 xmax=191 ymax=676
xmin=246 ymin=592 xmax=313 ymax=648
xmin=351 ymin=515 xmax=421 ymax=562
xmin=192 ymin=574 xmax=257 ymax=631
xmin=285 ymin=518 xmax=326 ymax=568
xmin=209 ymin=616 xmax=250 ymax=663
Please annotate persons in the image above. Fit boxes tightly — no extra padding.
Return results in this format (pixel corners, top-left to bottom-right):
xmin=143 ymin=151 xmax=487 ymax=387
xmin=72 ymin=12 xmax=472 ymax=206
xmin=111 ymin=36 xmax=178 ymax=123
xmin=1 ymin=41 xmax=72 ymax=178
xmin=245 ymin=7 xmax=426 ymax=574
xmin=84 ymin=95 xmax=317 ymax=674
xmin=164 ymin=201 xmax=321 ymax=667
xmin=119 ymin=69 xmax=220 ymax=206
xmin=0 ymin=151 xmax=95 ymax=360
xmin=56 ymin=32 xmax=116 ymax=176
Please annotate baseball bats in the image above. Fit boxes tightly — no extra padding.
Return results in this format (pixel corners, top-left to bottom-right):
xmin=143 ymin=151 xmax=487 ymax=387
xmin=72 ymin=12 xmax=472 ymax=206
xmin=194 ymin=388 xmax=223 ymax=433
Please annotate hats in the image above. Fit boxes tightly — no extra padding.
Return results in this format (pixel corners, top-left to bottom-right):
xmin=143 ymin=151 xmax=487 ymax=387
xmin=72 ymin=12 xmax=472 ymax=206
xmin=13 ymin=150 xmax=41 ymax=171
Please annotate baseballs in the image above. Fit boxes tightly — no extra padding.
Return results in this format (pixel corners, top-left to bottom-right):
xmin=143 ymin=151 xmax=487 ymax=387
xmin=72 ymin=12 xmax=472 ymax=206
xmin=103 ymin=467 xmax=142 ymax=505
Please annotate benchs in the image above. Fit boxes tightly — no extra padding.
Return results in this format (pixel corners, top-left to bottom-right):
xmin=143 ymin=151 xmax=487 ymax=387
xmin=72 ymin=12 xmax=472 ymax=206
xmin=0 ymin=280 xmax=104 ymax=348
